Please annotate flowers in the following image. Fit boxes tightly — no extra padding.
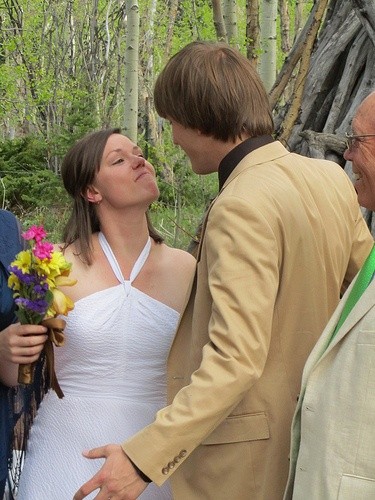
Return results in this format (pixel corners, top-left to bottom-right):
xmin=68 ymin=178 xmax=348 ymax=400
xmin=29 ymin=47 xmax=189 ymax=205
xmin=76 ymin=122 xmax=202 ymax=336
xmin=7 ymin=225 xmax=78 ymax=452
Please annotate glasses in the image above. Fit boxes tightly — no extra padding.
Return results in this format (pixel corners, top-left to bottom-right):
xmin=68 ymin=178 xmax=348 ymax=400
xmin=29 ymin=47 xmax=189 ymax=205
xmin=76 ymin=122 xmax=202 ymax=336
xmin=344 ymin=132 xmax=375 ymax=149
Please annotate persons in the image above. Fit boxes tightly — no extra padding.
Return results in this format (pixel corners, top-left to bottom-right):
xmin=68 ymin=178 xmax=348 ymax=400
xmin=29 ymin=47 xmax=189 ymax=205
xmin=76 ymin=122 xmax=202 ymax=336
xmin=0 ymin=126 xmax=200 ymax=500
xmin=281 ymin=91 xmax=375 ymax=500
xmin=71 ymin=41 xmax=375 ymax=500
